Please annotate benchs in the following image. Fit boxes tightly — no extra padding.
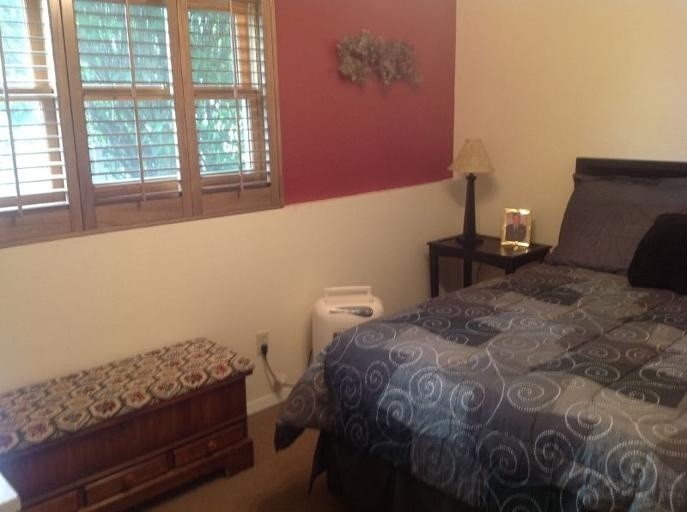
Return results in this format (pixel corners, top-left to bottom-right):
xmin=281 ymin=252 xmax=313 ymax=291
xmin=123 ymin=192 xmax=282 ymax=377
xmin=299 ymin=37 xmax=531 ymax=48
xmin=0 ymin=338 xmax=253 ymax=510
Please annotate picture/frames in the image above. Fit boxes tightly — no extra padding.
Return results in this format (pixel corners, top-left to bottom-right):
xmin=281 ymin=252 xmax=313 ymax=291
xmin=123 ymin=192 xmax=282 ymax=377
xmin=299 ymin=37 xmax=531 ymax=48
xmin=500 ymin=207 xmax=532 ymax=248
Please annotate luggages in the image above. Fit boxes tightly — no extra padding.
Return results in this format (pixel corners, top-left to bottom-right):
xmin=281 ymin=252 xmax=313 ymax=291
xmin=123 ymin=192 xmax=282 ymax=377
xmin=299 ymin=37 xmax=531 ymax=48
xmin=312 ymin=286 xmax=383 ymax=357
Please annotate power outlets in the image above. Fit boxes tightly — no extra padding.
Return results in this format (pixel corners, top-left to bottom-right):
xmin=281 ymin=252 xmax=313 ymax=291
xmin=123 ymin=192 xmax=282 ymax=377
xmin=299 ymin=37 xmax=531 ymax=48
xmin=255 ymin=331 xmax=270 ymax=357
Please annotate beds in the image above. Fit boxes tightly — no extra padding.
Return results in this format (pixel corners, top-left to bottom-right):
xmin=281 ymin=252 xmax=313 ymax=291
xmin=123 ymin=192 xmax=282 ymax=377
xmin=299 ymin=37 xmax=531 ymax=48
xmin=323 ymin=156 xmax=687 ymax=510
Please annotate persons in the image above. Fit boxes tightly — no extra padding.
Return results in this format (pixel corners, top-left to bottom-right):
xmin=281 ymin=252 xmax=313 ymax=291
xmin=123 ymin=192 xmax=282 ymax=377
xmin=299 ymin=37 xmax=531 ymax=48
xmin=506 ymin=213 xmax=527 ymax=243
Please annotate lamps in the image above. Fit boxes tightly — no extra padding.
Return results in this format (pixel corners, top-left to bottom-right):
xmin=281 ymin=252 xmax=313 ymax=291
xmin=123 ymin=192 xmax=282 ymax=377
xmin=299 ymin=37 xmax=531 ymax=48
xmin=447 ymin=137 xmax=494 ymax=245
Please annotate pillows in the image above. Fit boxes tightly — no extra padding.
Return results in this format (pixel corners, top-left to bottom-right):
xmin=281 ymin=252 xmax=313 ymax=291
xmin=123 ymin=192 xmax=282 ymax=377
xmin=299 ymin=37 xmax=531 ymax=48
xmin=541 ymin=172 xmax=686 ymax=295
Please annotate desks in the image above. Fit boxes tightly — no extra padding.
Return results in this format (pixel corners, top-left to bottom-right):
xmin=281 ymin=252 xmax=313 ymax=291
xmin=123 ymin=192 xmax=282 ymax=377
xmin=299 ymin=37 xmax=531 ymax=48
xmin=425 ymin=233 xmax=552 ymax=298
